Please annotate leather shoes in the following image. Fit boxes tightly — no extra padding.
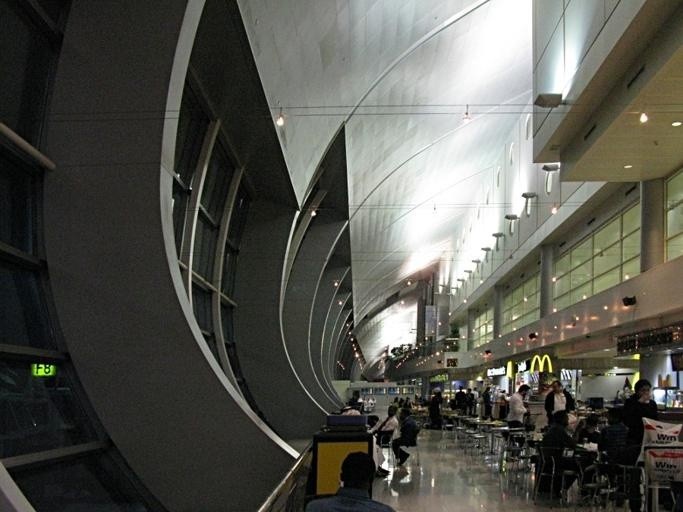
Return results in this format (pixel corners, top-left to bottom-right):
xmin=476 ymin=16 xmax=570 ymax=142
xmin=396 ymin=454 xmax=409 ymax=464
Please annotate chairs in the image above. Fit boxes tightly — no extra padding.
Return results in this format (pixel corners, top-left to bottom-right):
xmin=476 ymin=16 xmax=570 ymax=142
xmin=441 ymin=401 xmax=677 ymax=512
xmin=368 ymin=415 xmax=421 ymax=469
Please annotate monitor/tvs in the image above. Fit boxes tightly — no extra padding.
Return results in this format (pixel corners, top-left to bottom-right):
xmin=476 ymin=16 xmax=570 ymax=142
xmin=671 ymin=352 xmax=683 ymax=371
xmin=590 ymin=397 xmax=603 ymax=411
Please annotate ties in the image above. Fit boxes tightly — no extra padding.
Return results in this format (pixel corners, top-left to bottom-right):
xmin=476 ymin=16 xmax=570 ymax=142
xmin=378 ymin=417 xmax=390 ymax=431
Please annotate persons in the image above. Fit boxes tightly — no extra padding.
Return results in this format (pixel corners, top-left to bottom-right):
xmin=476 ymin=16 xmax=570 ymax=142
xmin=305 ymin=451 xmax=396 ymax=511
xmin=395 ymin=392 xmax=443 ymax=429
xmin=622 ymin=379 xmax=659 ymax=512
xmin=392 ymin=409 xmax=420 ymax=465
xmin=371 ymin=405 xmax=398 ymax=477
xmin=544 ymin=380 xmax=576 ymax=426
xmin=529 ymin=407 xmax=628 ymax=507
xmin=455 ymin=386 xmax=493 ymax=420
xmin=508 ymin=384 xmax=531 ymax=447
